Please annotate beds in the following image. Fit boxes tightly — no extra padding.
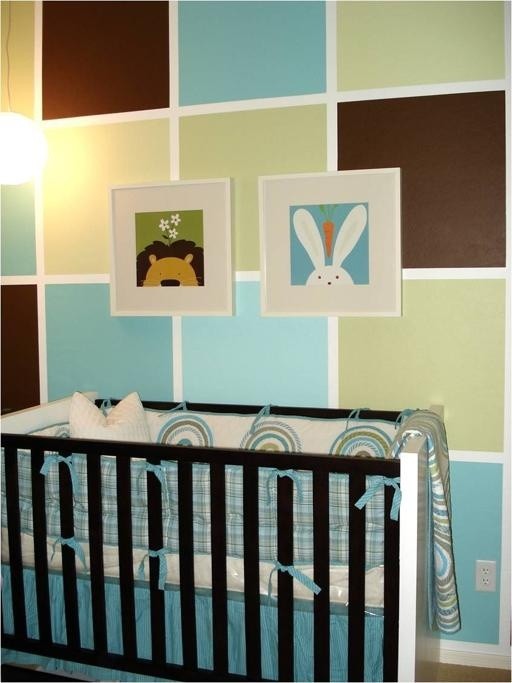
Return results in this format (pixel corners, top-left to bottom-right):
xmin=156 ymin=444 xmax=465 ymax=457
xmin=0 ymin=391 xmax=447 ymax=682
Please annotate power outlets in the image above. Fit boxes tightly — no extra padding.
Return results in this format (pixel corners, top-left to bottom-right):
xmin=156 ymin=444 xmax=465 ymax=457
xmin=476 ymin=559 xmax=497 ymax=592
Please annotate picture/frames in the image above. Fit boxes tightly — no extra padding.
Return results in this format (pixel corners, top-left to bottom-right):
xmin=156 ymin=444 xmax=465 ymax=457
xmin=257 ymin=167 xmax=404 ymax=318
xmin=107 ymin=178 xmax=235 ymax=318
